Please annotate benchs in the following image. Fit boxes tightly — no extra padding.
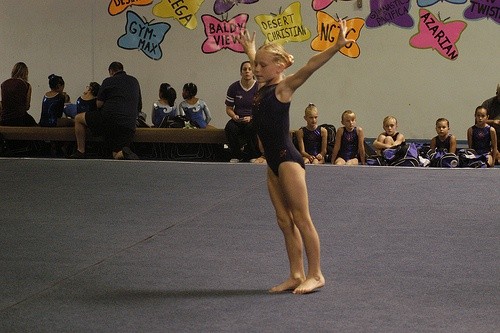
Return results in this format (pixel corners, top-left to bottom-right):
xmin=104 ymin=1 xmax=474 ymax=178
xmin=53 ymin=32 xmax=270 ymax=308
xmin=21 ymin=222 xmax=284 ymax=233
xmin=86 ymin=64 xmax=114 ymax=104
xmin=0 ymin=127 xmax=230 ymax=143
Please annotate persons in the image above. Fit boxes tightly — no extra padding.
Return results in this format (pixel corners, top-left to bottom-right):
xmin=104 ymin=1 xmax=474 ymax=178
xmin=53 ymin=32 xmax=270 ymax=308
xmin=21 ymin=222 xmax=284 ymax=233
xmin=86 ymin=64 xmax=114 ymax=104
xmin=0 ymin=62 xmax=38 ymax=127
xmin=331 ymin=110 xmax=365 ymax=166
xmin=467 ymin=106 xmax=497 ymax=167
xmin=216 ymin=61 xmax=267 ymax=163
xmin=77 ymin=82 xmax=100 ymax=114
xmin=39 ymin=74 xmax=75 ymax=126
xmin=151 ymin=83 xmax=179 ymax=126
xmin=298 ymin=104 xmax=327 ymax=164
xmin=65 ymin=61 xmax=142 ymax=159
xmin=373 ymin=116 xmax=406 ymax=157
xmin=431 ymin=118 xmax=457 ymax=156
xmin=481 ymin=83 xmax=500 ymax=164
xmin=236 ymin=19 xmax=355 ymax=294
xmin=179 ymin=83 xmax=212 ymax=129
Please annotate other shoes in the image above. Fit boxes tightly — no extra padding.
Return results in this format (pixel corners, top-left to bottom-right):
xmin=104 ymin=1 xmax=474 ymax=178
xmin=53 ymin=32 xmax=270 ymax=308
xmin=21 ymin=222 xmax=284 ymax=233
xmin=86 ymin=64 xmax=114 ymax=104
xmin=121 ymin=147 xmax=139 ymax=160
xmin=64 ymin=150 xmax=87 ymax=159
